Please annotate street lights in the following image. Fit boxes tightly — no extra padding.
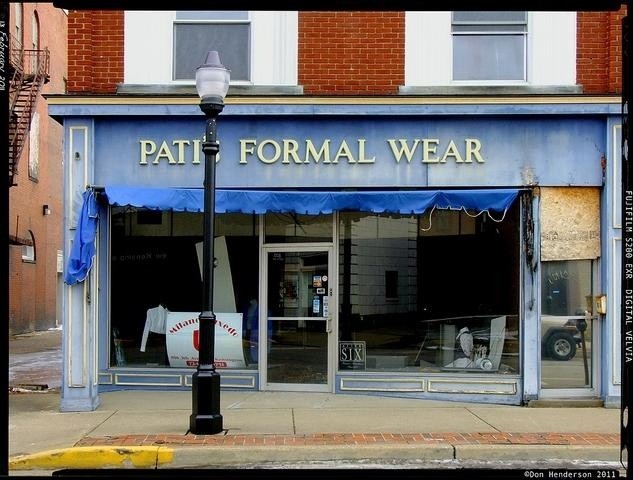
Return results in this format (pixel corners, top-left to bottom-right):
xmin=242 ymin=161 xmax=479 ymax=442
xmin=184 ymin=50 xmax=232 ymax=435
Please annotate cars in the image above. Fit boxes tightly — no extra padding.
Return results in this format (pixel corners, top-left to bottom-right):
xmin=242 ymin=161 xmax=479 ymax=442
xmin=466 ymin=314 xmax=593 ymax=360
